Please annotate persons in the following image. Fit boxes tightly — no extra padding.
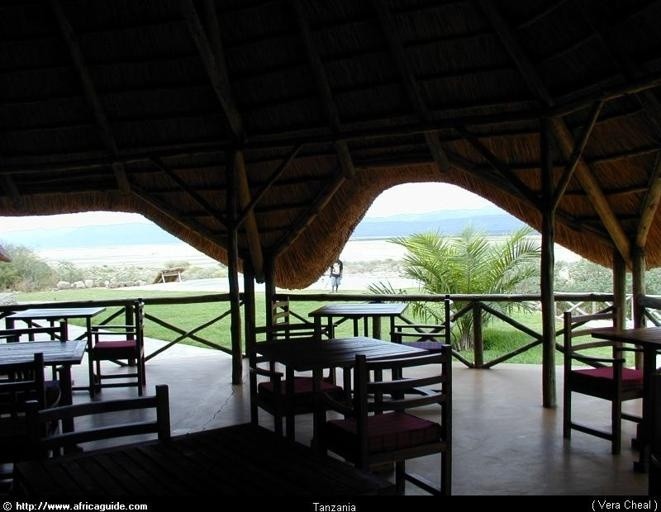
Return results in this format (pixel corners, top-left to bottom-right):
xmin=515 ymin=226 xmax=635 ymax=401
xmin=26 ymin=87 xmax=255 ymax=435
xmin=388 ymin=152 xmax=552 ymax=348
xmin=329 ymin=259 xmax=343 ymax=293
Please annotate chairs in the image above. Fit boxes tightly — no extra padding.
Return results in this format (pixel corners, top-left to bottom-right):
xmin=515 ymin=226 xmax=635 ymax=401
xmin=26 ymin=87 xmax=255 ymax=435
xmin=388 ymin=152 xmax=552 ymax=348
xmin=0 ymin=297 xmax=661 ymax=512
